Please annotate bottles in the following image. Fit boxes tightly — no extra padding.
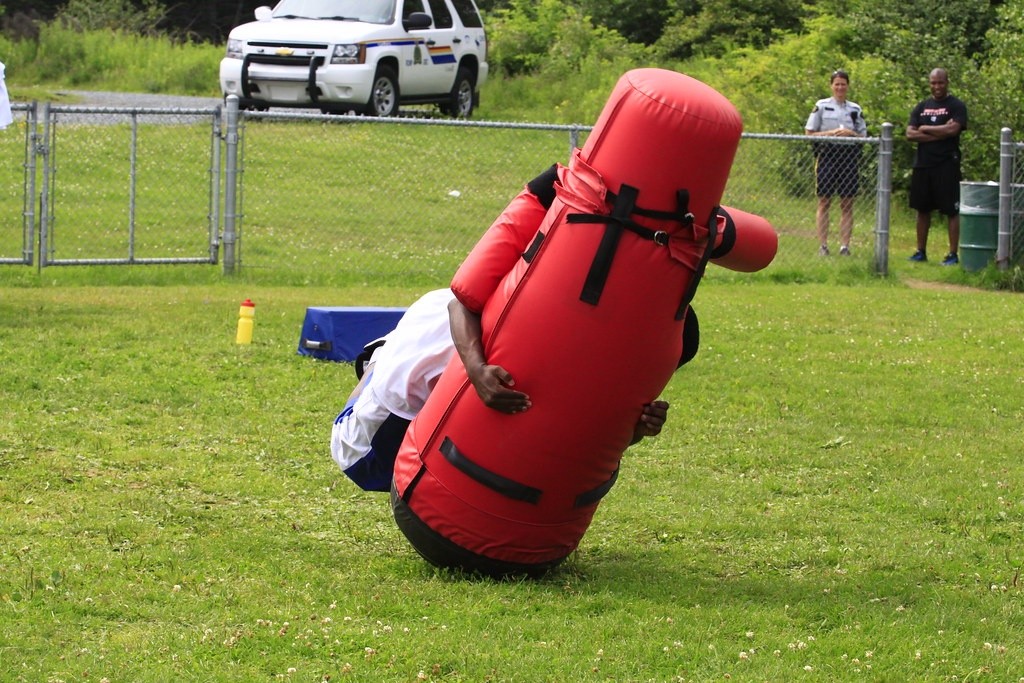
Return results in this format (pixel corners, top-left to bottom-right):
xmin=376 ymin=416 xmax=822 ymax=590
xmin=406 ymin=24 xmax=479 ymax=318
xmin=235 ymin=298 xmax=257 ymax=346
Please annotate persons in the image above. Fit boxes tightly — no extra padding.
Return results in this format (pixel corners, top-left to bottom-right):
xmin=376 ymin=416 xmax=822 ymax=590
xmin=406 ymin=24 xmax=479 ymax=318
xmin=329 ymin=162 xmax=699 ymax=491
xmin=804 ymin=72 xmax=867 ymax=255
xmin=906 ymin=67 xmax=969 ymax=265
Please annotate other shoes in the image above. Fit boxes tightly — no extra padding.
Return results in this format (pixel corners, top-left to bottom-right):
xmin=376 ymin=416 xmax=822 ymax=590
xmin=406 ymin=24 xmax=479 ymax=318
xmin=941 ymin=252 xmax=958 ymax=266
xmin=818 ymin=245 xmax=829 ymax=257
xmin=840 ymin=248 xmax=850 ymax=256
xmin=355 ymin=330 xmax=393 ymax=380
xmin=907 ymin=249 xmax=926 ymax=261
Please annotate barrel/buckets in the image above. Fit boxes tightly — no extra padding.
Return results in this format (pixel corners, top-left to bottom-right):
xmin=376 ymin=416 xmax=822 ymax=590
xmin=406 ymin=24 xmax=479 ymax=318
xmin=958 ymin=181 xmax=1024 ymax=274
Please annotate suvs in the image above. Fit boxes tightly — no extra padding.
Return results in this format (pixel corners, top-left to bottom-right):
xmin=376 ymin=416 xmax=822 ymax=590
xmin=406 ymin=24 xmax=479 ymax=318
xmin=218 ymin=0 xmax=489 ymax=121
